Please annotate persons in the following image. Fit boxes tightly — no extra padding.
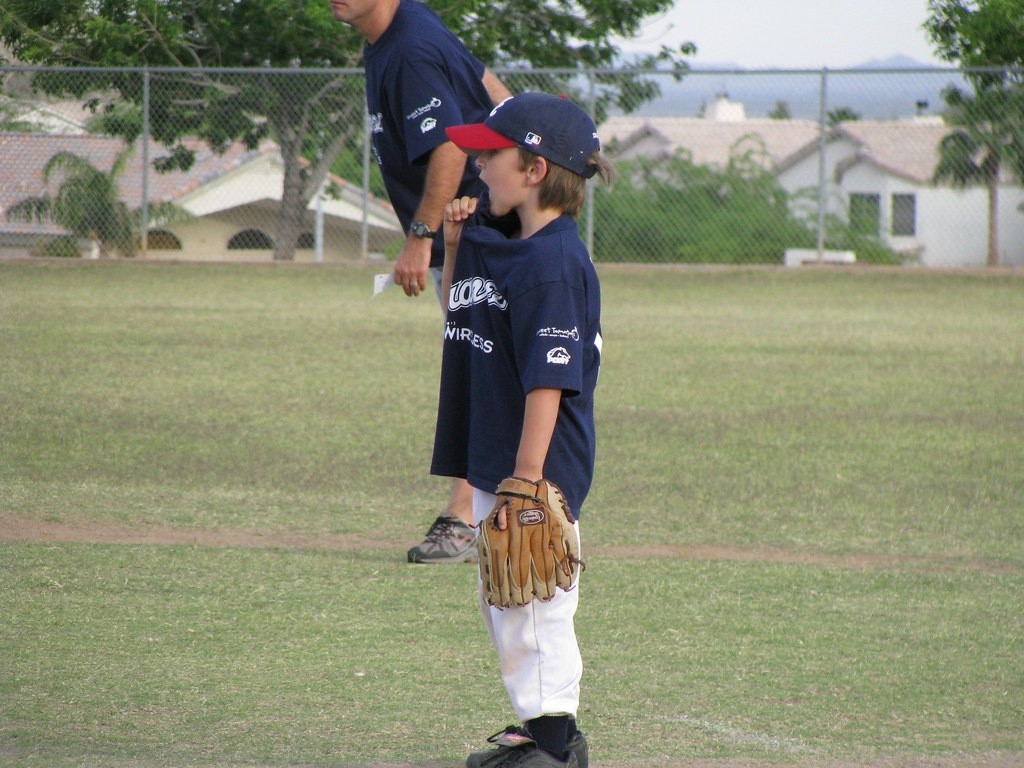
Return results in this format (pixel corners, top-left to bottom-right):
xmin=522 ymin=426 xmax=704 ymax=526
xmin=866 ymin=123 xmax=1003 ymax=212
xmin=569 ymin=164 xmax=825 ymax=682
xmin=327 ymin=1 xmax=522 ymax=565
xmin=427 ymin=91 xmax=602 ymax=767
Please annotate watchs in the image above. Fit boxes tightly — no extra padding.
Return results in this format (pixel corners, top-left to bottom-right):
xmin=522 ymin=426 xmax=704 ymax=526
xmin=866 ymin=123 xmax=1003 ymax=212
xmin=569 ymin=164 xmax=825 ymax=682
xmin=409 ymin=221 xmax=437 ymax=239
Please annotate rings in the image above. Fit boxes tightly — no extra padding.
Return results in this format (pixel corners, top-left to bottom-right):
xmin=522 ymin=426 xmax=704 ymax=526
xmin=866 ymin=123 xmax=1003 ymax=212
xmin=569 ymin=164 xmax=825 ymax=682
xmin=410 ymin=282 xmax=418 ymax=287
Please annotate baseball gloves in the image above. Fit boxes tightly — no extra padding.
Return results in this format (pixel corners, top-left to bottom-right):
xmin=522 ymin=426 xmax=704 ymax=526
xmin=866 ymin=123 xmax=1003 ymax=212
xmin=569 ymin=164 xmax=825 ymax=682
xmin=476 ymin=478 xmax=582 ymax=612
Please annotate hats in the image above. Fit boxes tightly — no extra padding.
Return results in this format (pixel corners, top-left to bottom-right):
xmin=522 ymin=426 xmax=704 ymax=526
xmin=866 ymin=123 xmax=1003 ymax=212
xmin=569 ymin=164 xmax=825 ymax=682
xmin=446 ymin=91 xmax=601 ymax=179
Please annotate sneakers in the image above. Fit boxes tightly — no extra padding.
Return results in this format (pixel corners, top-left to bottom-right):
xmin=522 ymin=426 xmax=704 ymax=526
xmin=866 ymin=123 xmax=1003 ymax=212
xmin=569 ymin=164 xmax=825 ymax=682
xmin=407 ymin=512 xmax=477 ymax=564
xmin=466 ymin=724 xmax=589 ymax=768
